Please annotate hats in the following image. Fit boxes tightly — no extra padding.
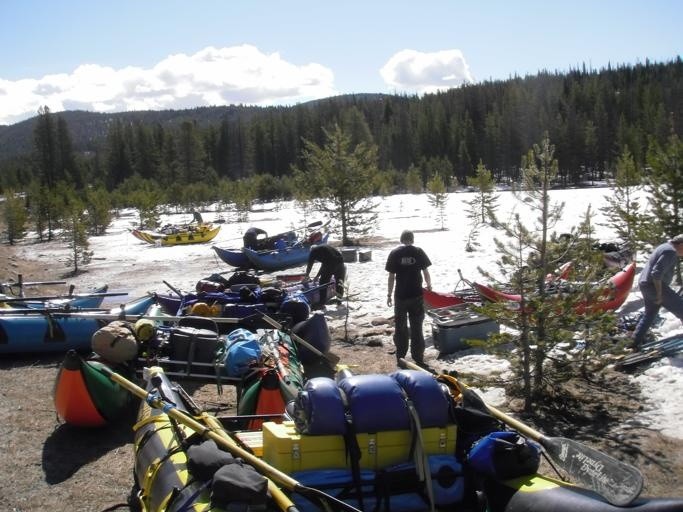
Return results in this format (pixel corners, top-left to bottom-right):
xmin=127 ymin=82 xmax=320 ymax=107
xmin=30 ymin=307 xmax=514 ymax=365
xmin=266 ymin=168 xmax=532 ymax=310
xmin=400 ymin=230 xmax=414 ymax=244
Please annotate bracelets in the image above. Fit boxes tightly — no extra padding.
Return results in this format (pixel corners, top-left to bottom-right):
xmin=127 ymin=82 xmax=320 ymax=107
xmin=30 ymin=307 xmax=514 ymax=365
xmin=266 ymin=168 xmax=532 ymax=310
xmin=386 ymin=294 xmax=391 ymax=297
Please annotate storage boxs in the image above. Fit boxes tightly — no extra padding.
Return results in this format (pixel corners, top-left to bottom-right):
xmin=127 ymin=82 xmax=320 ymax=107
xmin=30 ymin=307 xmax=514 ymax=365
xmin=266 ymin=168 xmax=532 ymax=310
xmin=342 ymin=249 xmax=357 ymax=262
xmin=431 ymin=311 xmax=495 ymax=353
xmin=170 ymin=326 xmax=217 ymax=374
xmin=359 ymin=250 xmax=371 ymax=262
xmin=262 ymin=419 xmax=457 ymax=474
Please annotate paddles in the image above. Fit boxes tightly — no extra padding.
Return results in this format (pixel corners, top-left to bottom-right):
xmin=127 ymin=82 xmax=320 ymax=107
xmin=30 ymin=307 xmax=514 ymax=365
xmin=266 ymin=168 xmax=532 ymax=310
xmin=254 ymin=309 xmax=340 ymax=366
xmin=293 ymin=219 xmax=331 ymax=246
xmin=258 ymin=221 xmax=322 ymax=244
xmin=210 ymin=220 xmax=225 ymax=223
xmin=53 ymin=312 xmax=287 ymax=334
xmin=399 ymin=357 xmax=644 ymax=506
xmin=100 ymin=366 xmax=362 ymax=512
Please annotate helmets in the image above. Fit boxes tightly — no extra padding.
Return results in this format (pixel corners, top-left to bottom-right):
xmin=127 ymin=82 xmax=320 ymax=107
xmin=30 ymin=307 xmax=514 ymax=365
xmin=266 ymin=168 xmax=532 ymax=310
xmin=309 ymin=231 xmax=322 ymax=243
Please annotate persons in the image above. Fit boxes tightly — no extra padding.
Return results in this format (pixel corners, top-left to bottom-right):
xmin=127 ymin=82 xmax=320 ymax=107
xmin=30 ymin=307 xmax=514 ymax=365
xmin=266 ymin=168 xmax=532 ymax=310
xmin=623 ymin=233 xmax=682 ymax=353
xmin=243 ymin=227 xmax=268 ymax=250
xmin=188 ymin=208 xmax=202 ymax=224
xmin=301 ymin=242 xmax=346 ymax=311
xmin=384 ymin=229 xmax=432 ymax=368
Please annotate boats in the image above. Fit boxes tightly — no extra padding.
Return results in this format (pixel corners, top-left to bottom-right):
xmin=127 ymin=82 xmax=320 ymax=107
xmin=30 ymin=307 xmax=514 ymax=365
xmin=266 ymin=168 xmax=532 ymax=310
xmin=126 ymin=222 xmax=221 ymax=246
xmin=212 ymin=232 xmax=300 ymax=268
xmin=242 ymin=231 xmax=331 ymax=270
